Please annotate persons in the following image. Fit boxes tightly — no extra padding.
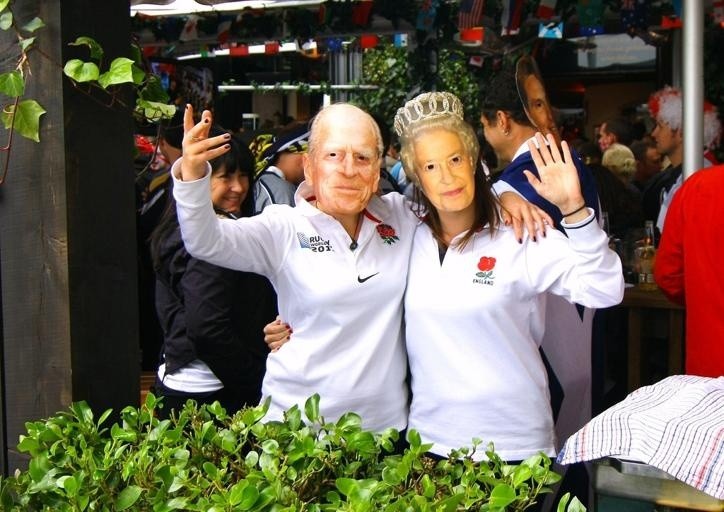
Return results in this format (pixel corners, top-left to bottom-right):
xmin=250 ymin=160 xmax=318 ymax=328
xmin=514 ymin=57 xmax=566 ymax=149
xmin=263 ymin=92 xmax=624 ymax=470
xmin=131 ymin=67 xmax=313 ymax=423
xmin=170 ymin=103 xmax=553 ymax=468
xmin=480 ymin=69 xmax=723 ymax=512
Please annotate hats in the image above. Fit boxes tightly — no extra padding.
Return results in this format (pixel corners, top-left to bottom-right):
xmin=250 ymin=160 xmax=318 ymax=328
xmin=648 ymin=89 xmax=720 ymax=145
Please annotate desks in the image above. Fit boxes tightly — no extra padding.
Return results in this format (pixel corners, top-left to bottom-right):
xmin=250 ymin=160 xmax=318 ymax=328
xmin=622 ymin=288 xmax=685 ymax=398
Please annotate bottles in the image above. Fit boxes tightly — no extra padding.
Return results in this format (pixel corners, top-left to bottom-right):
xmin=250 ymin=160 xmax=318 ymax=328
xmin=637 ymin=221 xmax=657 ymax=292
